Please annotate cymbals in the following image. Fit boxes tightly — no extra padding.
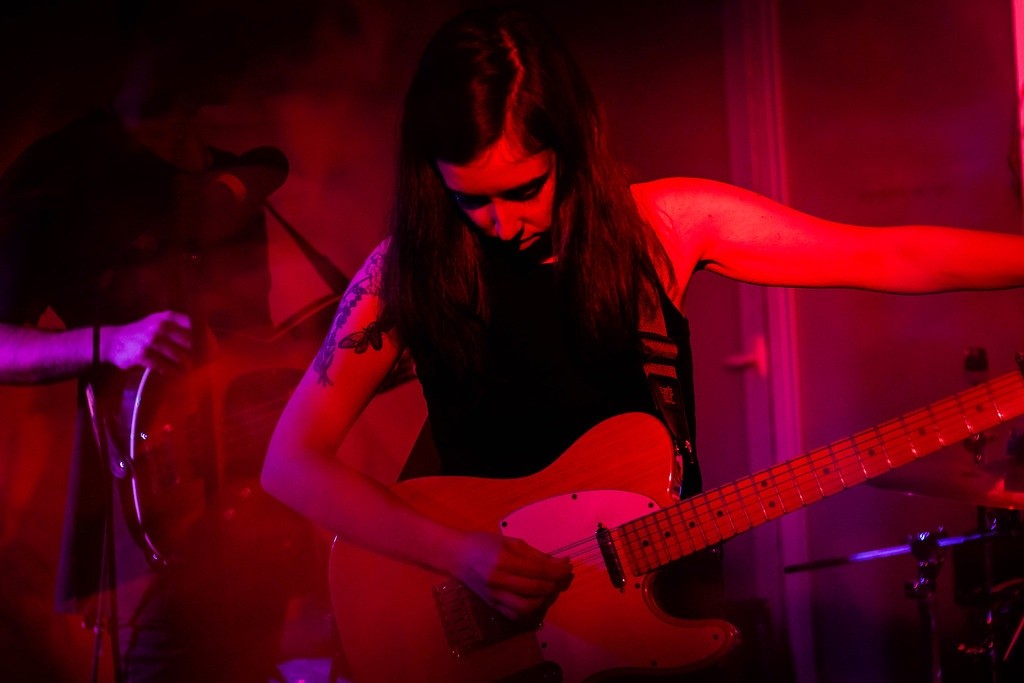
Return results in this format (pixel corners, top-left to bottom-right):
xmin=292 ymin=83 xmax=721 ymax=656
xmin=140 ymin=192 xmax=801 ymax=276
xmin=865 ymin=456 xmax=1024 ymax=513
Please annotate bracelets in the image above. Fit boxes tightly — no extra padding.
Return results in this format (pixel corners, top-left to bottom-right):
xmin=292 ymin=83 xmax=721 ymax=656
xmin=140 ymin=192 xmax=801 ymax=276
xmin=93 ymin=324 xmax=102 ymax=363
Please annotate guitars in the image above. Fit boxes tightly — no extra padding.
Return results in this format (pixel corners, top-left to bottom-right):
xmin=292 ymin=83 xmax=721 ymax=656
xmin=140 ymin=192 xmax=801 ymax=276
xmin=116 ymin=288 xmax=345 ymax=576
xmin=323 ymin=367 xmax=1024 ymax=683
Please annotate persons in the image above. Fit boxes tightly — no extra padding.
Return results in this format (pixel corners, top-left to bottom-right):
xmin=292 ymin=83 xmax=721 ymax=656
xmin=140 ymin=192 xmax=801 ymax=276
xmin=0 ymin=0 xmax=272 ymax=605
xmin=258 ymin=24 xmax=1024 ymax=620
